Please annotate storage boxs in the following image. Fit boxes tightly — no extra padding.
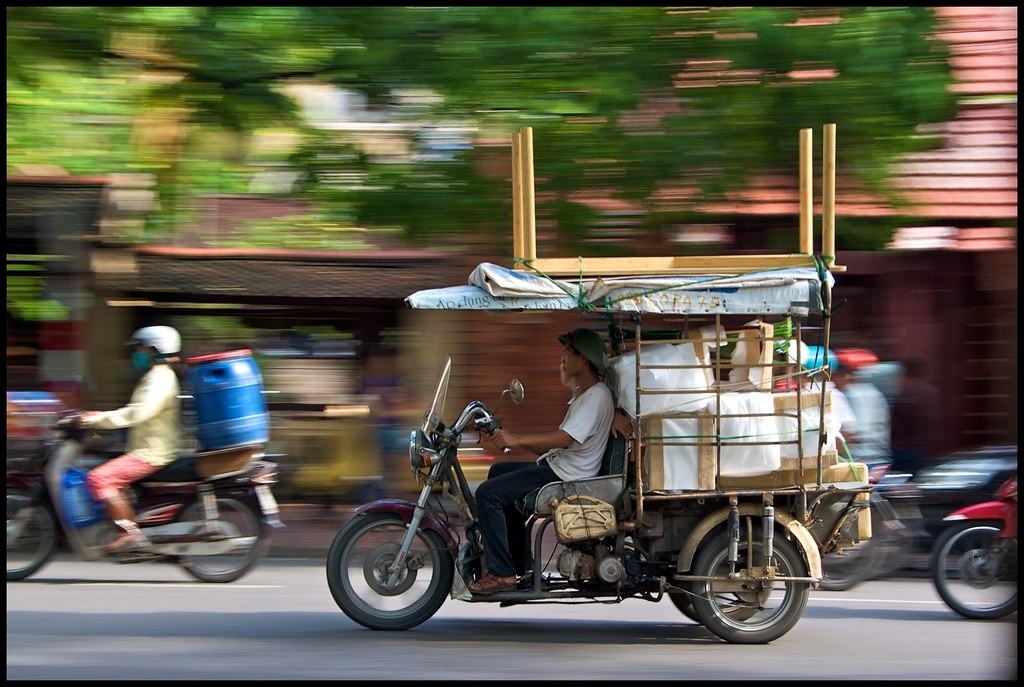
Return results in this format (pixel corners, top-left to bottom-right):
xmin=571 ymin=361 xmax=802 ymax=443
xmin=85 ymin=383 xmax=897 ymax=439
xmin=614 ymin=322 xmax=872 ymax=542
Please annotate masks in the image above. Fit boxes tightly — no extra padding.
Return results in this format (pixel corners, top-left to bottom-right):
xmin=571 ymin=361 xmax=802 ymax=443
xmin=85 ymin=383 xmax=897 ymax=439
xmin=131 ymin=349 xmax=160 ymax=367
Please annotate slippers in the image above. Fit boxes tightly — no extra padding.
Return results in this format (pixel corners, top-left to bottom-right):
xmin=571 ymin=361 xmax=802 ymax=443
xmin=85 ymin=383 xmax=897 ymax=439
xmin=469 ymin=573 xmax=518 ymax=594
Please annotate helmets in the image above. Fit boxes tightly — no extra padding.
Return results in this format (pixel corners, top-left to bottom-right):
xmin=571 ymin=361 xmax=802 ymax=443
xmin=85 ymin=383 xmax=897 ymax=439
xmin=801 ymin=345 xmax=840 ymax=376
xmin=833 ymin=346 xmax=878 ymax=371
xmin=773 ymin=338 xmax=809 ymax=365
xmin=123 ymin=326 xmax=182 ymax=354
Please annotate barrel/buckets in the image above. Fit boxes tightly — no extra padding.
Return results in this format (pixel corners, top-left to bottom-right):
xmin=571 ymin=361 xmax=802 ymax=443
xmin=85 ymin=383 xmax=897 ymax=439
xmin=186 ymin=349 xmax=270 ymax=451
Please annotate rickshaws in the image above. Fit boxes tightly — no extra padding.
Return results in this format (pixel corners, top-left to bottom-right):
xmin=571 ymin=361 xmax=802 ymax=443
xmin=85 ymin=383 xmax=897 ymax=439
xmin=324 ymin=275 xmax=873 ymax=643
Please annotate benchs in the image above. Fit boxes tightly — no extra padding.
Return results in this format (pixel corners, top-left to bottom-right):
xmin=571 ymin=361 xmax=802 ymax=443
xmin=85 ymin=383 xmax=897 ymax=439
xmin=524 ymin=409 xmax=630 ymax=514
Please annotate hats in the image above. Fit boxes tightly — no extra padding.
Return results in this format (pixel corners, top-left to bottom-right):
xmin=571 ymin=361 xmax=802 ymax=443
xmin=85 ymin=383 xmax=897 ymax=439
xmin=558 ymin=328 xmax=608 ymax=377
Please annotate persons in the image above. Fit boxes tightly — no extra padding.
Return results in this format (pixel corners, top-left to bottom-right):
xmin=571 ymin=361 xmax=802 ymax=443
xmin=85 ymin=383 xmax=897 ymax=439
xmin=78 ymin=325 xmax=181 ymax=554
xmin=774 ymin=337 xmax=893 ymax=469
xmin=469 ymin=329 xmax=635 ymax=595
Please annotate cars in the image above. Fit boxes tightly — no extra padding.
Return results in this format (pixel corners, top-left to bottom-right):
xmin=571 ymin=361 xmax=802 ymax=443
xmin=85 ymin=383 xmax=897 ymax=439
xmin=880 ymin=446 xmax=1021 ymax=567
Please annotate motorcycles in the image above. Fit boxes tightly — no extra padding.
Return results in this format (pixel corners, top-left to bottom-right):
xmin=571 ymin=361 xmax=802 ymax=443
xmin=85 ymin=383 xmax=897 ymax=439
xmin=930 ymin=474 xmax=1020 ymax=621
xmin=5 ymin=418 xmax=288 ymax=584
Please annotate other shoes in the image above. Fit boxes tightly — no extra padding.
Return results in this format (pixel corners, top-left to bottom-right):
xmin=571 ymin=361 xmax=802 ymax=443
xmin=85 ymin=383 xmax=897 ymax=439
xmin=104 ymin=532 xmax=149 ymax=552
